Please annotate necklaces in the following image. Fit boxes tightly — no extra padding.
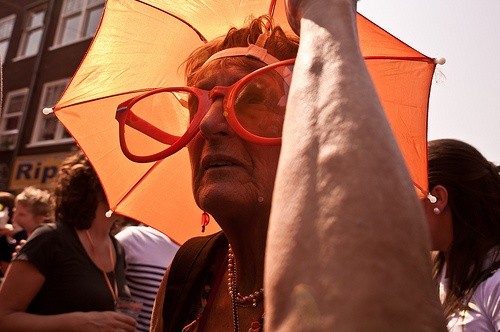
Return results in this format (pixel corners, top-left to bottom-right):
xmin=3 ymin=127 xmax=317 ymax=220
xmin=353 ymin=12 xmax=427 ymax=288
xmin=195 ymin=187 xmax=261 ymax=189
xmin=86 ymin=229 xmax=119 ymax=307
xmin=227 ymin=243 xmax=264 ymax=332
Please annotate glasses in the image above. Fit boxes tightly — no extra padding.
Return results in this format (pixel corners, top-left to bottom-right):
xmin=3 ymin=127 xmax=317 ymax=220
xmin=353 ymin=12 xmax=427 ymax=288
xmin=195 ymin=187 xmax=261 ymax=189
xmin=115 ymin=57 xmax=296 ymax=163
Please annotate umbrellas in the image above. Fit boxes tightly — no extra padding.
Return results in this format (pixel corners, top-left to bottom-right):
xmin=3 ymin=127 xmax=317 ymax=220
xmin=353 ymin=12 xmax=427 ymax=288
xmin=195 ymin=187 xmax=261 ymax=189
xmin=43 ymin=0 xmax=446 ymax=246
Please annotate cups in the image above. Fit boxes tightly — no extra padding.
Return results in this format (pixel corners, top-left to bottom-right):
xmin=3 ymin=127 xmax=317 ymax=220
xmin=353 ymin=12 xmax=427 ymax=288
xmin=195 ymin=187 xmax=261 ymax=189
xmin=115 ymin=296 xmax=144 ymax=322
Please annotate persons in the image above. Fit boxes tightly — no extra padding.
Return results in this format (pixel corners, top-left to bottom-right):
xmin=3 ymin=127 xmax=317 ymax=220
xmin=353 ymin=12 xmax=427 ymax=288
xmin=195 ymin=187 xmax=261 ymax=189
xmin=149 ymin=0 xmax=448 ymax=332
xmin=0 ymin=153 xmax=181 ymax=332
xmin=423 ymin=138 xmax=500 ymax=332
